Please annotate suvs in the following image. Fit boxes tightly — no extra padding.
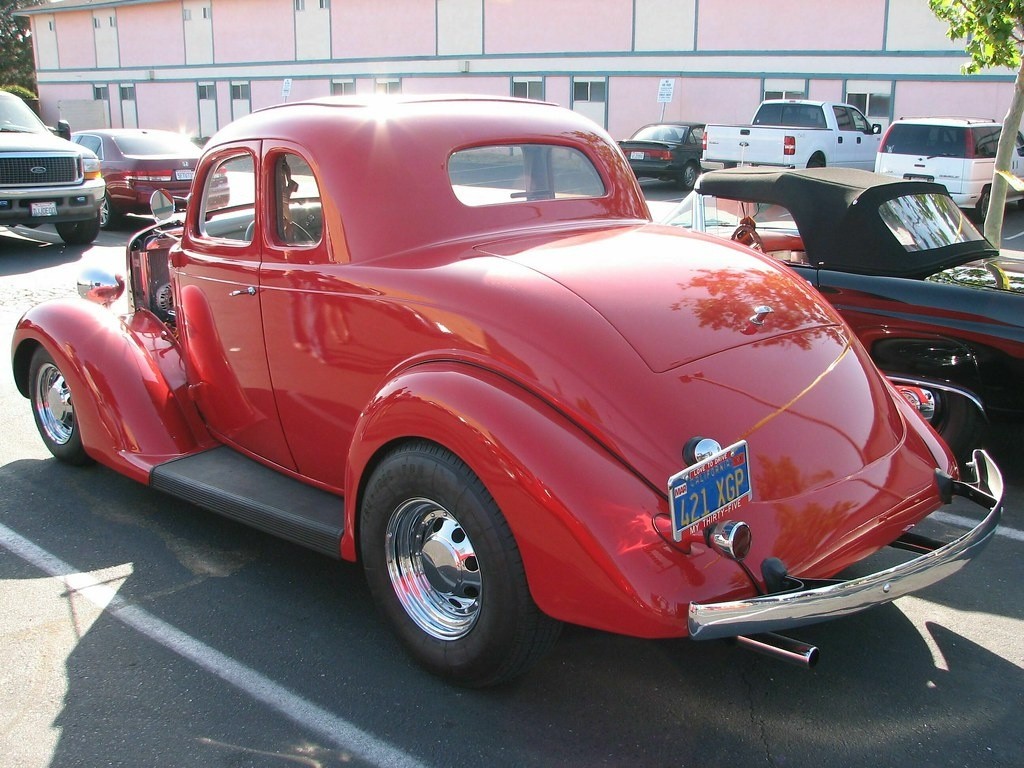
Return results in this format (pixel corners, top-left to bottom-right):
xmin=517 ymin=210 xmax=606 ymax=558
xmin=0 ymin=90 xmax=106 ymax=243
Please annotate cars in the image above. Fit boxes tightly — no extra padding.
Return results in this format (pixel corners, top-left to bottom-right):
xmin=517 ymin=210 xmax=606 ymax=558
xmin=657 ymin=161 xmax=1024 ymax=477
xmin=70 ymin=125 xmax=231 ymax=231
xmin=7 ymin=87 xmax=1008 ymax=693
xmin=615 ymin=120 xmax=706 ymax=192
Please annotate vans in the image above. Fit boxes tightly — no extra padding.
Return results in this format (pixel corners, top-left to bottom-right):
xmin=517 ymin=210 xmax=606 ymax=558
xmin=872 ymin=115 xmax=1024 ymax=224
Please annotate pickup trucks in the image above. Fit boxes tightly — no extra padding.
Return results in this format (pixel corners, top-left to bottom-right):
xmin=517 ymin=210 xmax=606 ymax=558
xmin=699 ymin=98 xmax=885 ymax=173
